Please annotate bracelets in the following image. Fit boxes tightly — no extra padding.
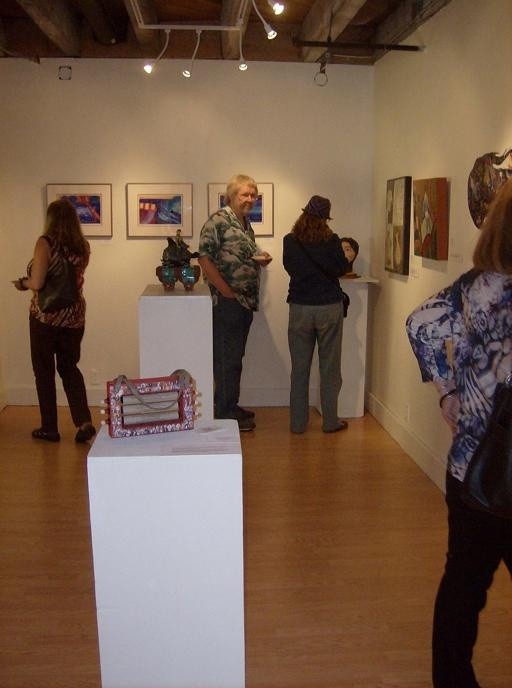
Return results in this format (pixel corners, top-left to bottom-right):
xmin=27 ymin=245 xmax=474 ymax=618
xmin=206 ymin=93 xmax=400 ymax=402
xmin=439 ymin=388 xmax=458 ymax=409
xmin=436 ymin=381 xmax=454 ymax=393
xmin=18 ymin=275 xmax=28 ymax=290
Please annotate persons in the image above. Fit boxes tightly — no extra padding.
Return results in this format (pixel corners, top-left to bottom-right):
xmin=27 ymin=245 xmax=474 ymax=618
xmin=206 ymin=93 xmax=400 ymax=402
xmin=403 ymin=176 xmax=512 ymax=687
xmin=7 ymin=197 xmax=96 ymax=442
xmin=199 ymin=173 xmax=273 ymax=431
xmin=339 ymin=236 xmax=360 ymax=265
xmin=282 ymin=194 xmax=352 ymax=435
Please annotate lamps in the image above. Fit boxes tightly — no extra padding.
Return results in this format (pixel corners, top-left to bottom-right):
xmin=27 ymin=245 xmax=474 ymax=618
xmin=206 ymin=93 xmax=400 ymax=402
xmin=131 ymin=0 xmax=284 ymax=79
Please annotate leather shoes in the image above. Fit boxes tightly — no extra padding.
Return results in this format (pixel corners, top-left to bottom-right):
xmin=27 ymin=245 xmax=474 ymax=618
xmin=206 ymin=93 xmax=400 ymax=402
xmin=75 ymin=425 xmax=96 ymax=442
xmin=323 ymin=421 xmax=347 ymax=432
xmin=237 ymin=408 xmax=255 ymax=431
xmin=32 ymin=429 xmax=61 ymax=441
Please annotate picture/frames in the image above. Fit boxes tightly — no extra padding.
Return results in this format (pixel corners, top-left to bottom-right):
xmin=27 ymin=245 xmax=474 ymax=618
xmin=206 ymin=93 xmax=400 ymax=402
xmin=205 ymin=182 xmax=275 ymax=238
xmin=45 ymin=182 xmax=112 ymax=240
xmin=125 ymin=181 xmax=195 ymax=240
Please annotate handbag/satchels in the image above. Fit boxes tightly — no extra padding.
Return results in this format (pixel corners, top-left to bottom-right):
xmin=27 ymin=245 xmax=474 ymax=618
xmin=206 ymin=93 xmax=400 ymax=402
xmin=100 ymin=370 xmax=203 ymax=438
xmin=38 ymin=235 xmax=79 ymax=313
xmin=460 ymin=374 xmax=511 ymax=519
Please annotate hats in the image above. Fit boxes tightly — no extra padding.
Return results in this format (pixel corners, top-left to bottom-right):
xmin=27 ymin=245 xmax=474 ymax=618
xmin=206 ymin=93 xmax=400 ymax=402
xmin=302 ymin=196 xmax=334 ymax=220
